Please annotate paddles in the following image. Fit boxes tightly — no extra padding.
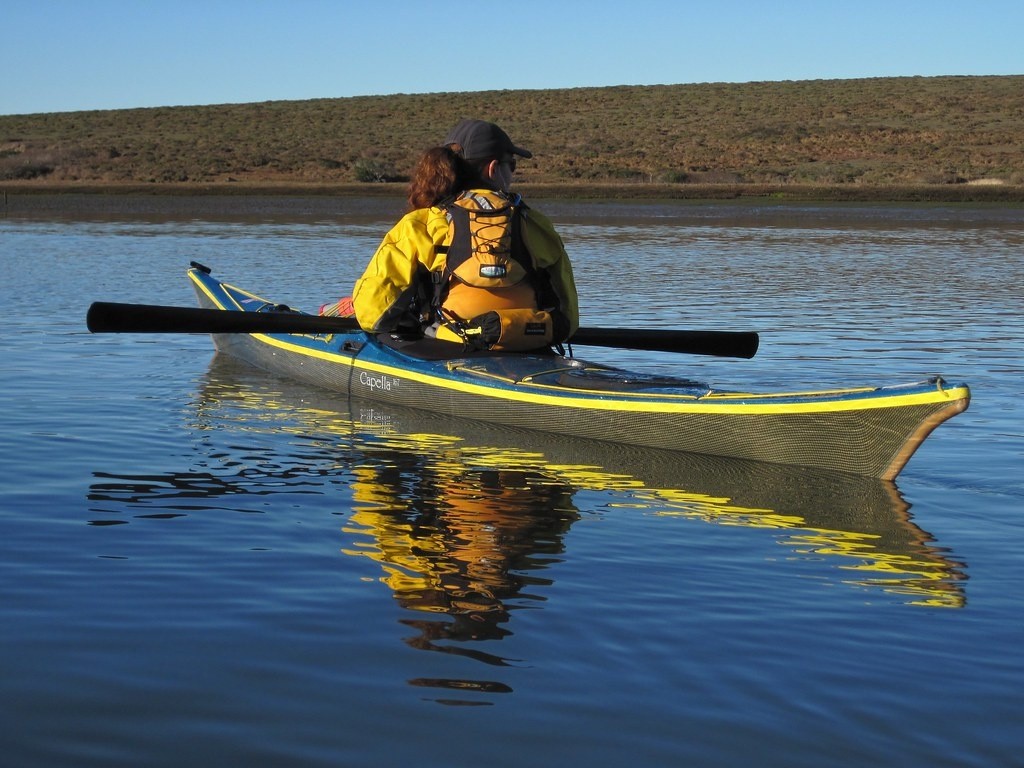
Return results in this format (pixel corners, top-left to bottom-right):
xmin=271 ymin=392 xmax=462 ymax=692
xmin=85 ymin=300 xmax=761 ymax=361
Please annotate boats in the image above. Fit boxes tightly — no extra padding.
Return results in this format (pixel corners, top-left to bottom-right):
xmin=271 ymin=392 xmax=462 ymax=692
xmin=189 ymin=350 xmax=968 ymax=611
xmin=188 ymin=260 xmax=968 ymax=482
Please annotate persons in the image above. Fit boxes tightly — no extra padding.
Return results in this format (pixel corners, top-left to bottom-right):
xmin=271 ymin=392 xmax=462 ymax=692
xmin=352 ymin=118 xmax=579 ymax=351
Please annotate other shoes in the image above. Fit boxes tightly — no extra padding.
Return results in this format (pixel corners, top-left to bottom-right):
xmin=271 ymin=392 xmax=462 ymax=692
xmin=320 ymin=296 xmax=354 ymax=316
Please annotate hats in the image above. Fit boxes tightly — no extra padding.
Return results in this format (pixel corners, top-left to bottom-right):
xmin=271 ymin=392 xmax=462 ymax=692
xmin=445 ymin=119 xmax=533 ymax=160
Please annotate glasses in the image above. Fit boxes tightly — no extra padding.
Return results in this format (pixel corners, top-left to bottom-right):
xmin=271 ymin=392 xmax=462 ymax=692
xmin=489 ymin=159 xmax=516 ymax=173
